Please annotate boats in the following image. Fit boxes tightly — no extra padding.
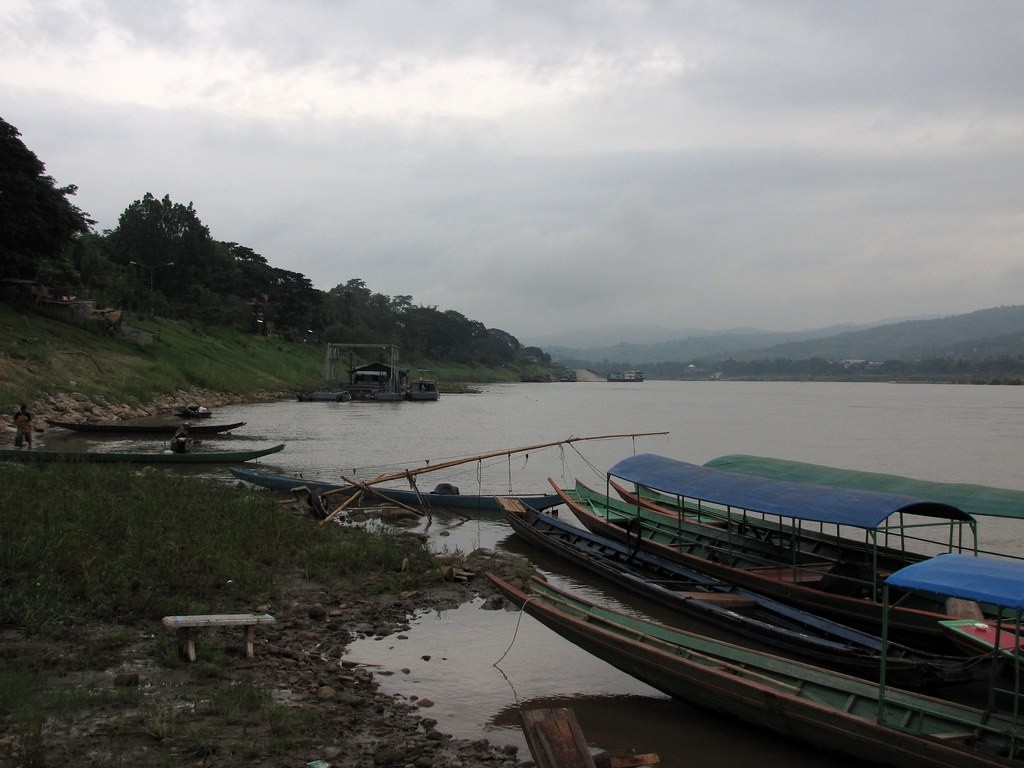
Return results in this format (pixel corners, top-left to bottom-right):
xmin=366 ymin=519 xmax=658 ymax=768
xmin=227 ymin=462 xmax=568 ymax=514
xmin=606 ymin=370 xmax=646 ymax=382
xmin=406 ymin=367 xmax=443 ymax=403
xmin=0 ymin=442 xmax=287 ymax=464
xmin=44 ymin=417 xmax=247 ymax=436
xmin=481 ymin=447 xmax=1024 ymax=768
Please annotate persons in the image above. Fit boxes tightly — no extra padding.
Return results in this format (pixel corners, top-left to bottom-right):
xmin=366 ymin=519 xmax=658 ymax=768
xmin=176 ymin=423 xmax=193 ymax=444
xmin=13 ymin=403 xmax=32 ymax=450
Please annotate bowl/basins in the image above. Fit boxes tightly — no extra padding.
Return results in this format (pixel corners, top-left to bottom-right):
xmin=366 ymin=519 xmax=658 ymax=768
xmin=975 ymin=623 xmax=988 ymax=631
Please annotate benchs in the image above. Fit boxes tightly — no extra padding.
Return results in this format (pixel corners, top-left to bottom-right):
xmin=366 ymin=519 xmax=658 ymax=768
xmin=162 ymin=614 xmax=276 ymax=661
